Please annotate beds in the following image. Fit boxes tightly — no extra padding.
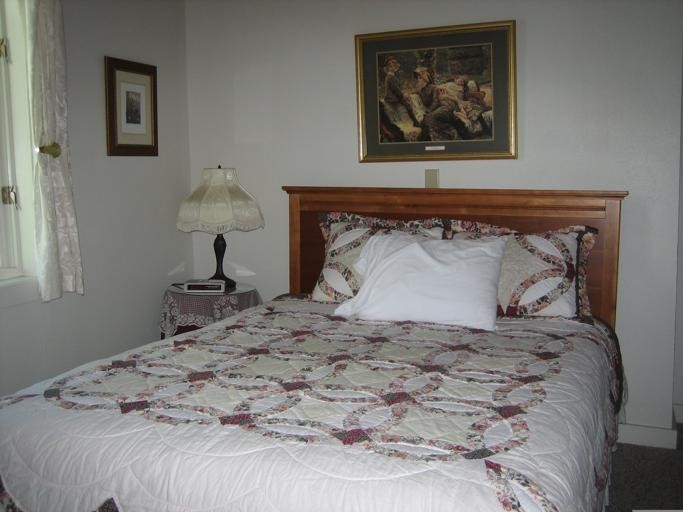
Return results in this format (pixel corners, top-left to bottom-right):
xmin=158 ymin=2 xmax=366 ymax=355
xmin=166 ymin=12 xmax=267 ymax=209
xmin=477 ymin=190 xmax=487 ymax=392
xmin=0 ymin=187 xmax=628 ymax=511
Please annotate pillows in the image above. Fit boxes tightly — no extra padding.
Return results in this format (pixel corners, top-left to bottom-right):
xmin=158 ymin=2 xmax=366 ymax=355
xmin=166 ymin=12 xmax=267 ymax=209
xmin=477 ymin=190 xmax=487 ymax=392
xmin=334 ymin=236 xmax=511 ymax=332
xmin=448 ymin=219 xmax=599 ymax=326
xmin=302 ymin=212 xmax=447 ymax=304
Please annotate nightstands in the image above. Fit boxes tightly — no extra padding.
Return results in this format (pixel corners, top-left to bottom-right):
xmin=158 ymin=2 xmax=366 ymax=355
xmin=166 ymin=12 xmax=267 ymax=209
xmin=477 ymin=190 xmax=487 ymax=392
xmin=158 ymin=282 xmax=262 ymax=339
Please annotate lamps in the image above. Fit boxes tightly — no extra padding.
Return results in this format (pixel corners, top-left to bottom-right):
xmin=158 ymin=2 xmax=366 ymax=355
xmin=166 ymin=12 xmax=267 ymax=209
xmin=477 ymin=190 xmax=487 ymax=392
xmin=176 ymin=165 xmax=263 ymax=288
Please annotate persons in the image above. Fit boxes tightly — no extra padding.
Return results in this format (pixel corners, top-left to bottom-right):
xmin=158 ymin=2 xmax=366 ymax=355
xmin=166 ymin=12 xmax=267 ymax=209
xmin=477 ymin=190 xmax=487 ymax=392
xmin=383 ymin=56 xmax=493 ymax=142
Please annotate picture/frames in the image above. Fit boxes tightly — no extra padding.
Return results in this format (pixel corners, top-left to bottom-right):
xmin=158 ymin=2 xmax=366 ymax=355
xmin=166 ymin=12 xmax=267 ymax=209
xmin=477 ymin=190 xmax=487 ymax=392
xmin=104 ymin=55 xmax=158 ymax=156
xmin=354 ymin=21 xmax=518 ymax=163
